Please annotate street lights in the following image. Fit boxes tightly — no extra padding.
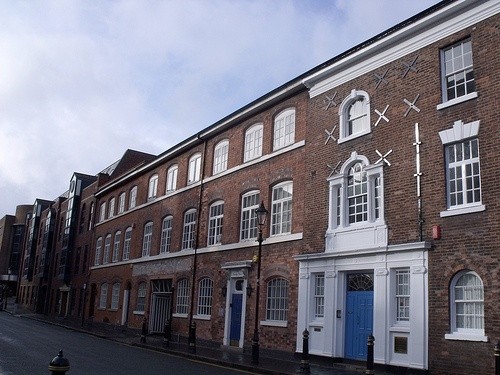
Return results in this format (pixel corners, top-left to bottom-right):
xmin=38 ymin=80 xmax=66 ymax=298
xmin=253 ymin=200 xmax=269 ymax=353
xmin=4 ymin=268 xmax=12 ymax=311
